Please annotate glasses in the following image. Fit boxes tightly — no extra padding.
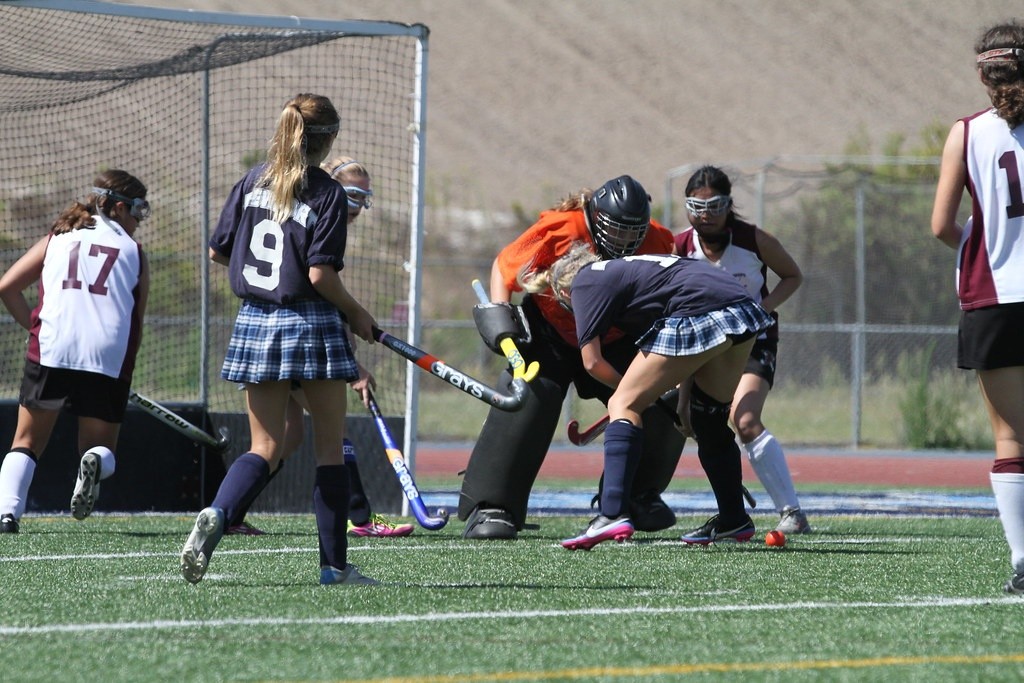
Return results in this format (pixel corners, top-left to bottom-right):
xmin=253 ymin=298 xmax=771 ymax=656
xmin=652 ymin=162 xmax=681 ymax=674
xmin=92 ymin=186 xmax=152 ymax=224
xmin=342 ymin=184 xmax=374 ymax=209
xmin=683 ymin=194 xmax=730 ymax=218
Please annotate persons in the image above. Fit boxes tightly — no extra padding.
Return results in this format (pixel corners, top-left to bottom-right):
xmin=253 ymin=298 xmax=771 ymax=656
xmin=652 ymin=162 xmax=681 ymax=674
xmin=221 ymin=153 xmax=414 ymax=539
xmin=514 ymin=238 xmax=814 ymax=549
xmin=0 ymin=168 xmax=152 ymax=532
xmin=179 ymin=92 xmax=382 ymax=584
xmin=454 ymin=172 xmax=689 ymax=540
xmin=672 ymin=165 xmax=812 ymax=533
xmin=929 ymin=22 xmax=1024 ymax=599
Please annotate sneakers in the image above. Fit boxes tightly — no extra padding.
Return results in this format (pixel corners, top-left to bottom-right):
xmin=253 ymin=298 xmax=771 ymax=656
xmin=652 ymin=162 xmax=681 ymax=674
xmin=680 ymin=514 xmax=757 ymax=547
xmin=320 ymin=563 xmax=380 ymax=587
xmin=999 ymin=556 xmax=1024 ymax=596
xmin=775 ymin=509 xmax=812 ymax=536
xmin=179 ymin=507 xmax=225 ymax=584
xmin=224 ymin=521 xmax=266 ymax=535
xmin=560 ymin=513 xmax=635 ymax=551
xmin=70 ymin=452 xmax=101 ymax=521
xmin=346 ymin=520 xmax=415 ymax=539
xmin=0 ymin=512 xmax=20 ymax=534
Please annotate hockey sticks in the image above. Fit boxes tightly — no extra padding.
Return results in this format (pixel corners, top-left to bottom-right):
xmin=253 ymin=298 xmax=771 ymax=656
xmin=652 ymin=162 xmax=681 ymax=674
xmin=365 ymin=391 xmax=450 ymax=531
xmin=374 ymin=278 xmax=541 ymax=412
xmin=567 ymin=380 xmax=680 ymax=446
xmin=129 ymin=389 xmax=233 ymax=453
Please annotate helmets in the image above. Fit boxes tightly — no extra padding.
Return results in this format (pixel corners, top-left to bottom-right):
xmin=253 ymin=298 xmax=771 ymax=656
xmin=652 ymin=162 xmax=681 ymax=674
xmin=588 ymin=174 xmax=651 ymax=263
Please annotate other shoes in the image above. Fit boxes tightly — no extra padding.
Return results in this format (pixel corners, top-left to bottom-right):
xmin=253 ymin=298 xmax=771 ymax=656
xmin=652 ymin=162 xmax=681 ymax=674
xmin=598 ymin=494 xmax=678 ymax=532
xmin=462 ymin=507 xmax=519 ymax=540
